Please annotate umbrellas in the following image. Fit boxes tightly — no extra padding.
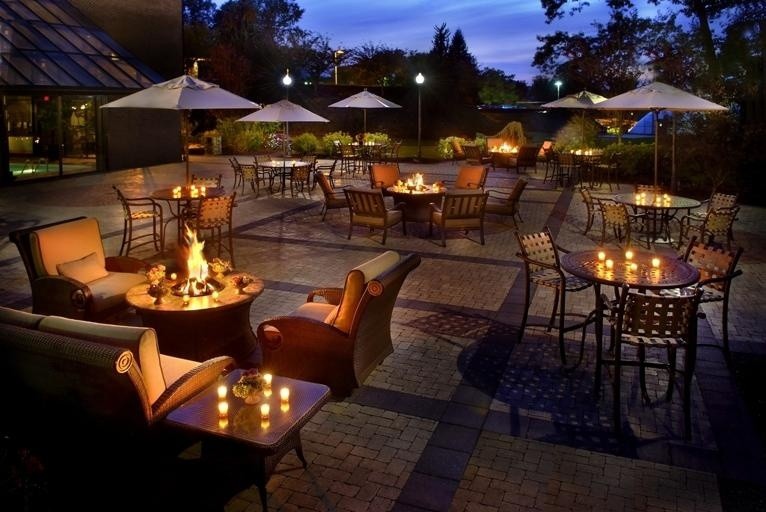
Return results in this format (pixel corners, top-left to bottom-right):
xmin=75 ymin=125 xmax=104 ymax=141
xmin=539 ymin=88 xmax=609 ymax=154
xmin=590 ymin=80 xmax=730 ymax=201
xmin=233 ymin=99 xmax=330 ymax=184
xmin=326 ymin=87 xmax=402 ymax=136
xmin=97 ymin=72 xmax=264 ymax=234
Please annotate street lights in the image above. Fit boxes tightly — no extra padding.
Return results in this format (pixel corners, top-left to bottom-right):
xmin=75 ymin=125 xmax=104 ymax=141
xmin=280 ymin=67 xmax=296 ymax=157
xmin=328 ymin=44 xmax=348 ymax=82
xmin=412 ymin=69 xmax=427 ymax=159
xmin=554 ymin=77 xmax=565 ymax=101
